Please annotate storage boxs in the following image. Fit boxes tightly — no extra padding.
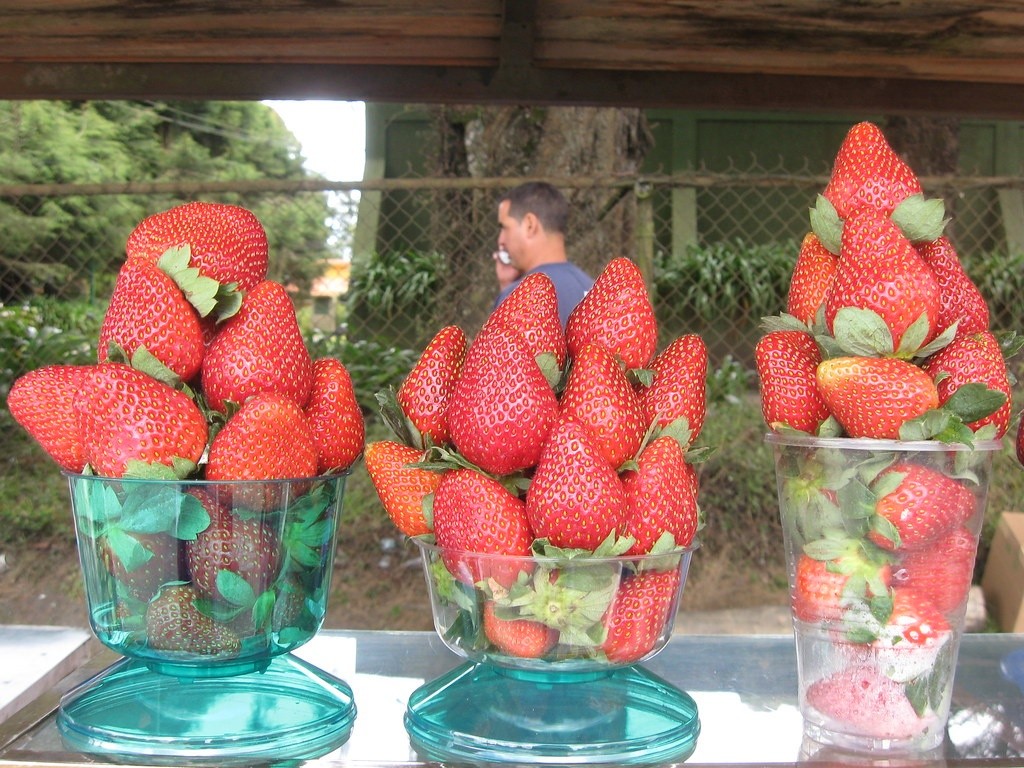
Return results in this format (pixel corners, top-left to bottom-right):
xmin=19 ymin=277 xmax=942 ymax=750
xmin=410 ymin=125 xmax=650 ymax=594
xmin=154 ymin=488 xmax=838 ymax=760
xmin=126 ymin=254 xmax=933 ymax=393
xmin=979 ymin=509 xmax=1024 ymax=632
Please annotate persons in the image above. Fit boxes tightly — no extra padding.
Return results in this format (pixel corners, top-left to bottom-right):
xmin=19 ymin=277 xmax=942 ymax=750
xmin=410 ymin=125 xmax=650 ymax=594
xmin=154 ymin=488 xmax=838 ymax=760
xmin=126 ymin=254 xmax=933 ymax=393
xmin=491 ymin=182 xmax=596 ymax=335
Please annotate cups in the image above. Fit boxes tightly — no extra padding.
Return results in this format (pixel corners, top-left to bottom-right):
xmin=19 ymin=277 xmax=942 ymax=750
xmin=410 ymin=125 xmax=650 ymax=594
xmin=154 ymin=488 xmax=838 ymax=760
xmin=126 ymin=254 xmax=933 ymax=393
xmin=766 ymin=431 xmax=1004 ymax=756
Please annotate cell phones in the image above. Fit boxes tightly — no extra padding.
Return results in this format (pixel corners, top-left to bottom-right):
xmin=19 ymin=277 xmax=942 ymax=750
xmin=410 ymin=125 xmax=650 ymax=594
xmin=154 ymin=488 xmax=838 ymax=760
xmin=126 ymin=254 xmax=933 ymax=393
xmin=498 ymin=251 xmax=510 ymax=265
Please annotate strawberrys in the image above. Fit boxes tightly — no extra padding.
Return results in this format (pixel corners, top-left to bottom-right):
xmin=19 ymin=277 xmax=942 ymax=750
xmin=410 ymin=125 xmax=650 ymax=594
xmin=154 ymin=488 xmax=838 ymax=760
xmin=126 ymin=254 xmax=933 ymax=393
xmin=756 ymin=122 xmax=1013 ymax=735
xmin=361 ymin=258 xmax=707 ymax=663
xmin=7 ymin=204 xmax=368 ymax=652
